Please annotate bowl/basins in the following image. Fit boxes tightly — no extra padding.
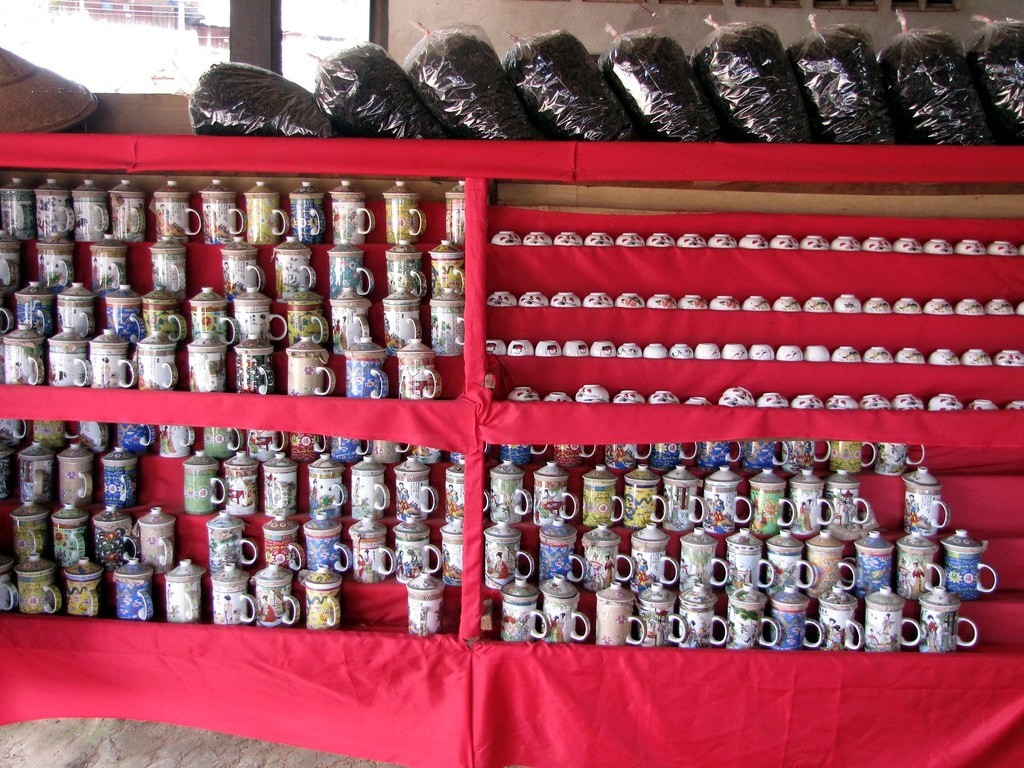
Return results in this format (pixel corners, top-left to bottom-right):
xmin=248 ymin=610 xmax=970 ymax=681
xmin=805 ymin=345 xmax=830 ymax=362
xmin=617 ymin=343 xmax=643 ymax=359
xmin=643 ymin=344 xmax=668 ymax=359
xmin=749 ymin=345 xmax=775 ymax=360
xmin=506 ymin=384 xmax=1024 ymax=409
xmin=492 ymin=230 xmax=1024 ymax=256
xmin=863 ymin=346 xmax=926 ymax=364
xmin=487 ymin=291 xmax=1024 ymax=315
xmin=669 ymin=344 xmax=694 ymax=359
xmin=562 ymin=340 xmax=589 ymax=356
xmin=722 ymin=344 xmax=748 ymax=360
xmin=776 ymin=345 xmax=803 ymax=361
xmin=535 ymin=340 xmax=561 ymax=357
xmin=590 ymin=341 xmax=616 ymax=357
xmin=507 ymin=340 xmax=534 ymax=356
xmin=695 ymin=343 xmax=720 ymax=360
xmin=486 ymin=339 xmax=507 ymax=355
xmin=831 ymin=347 xmax=862 ymax=362
xmin=928 ymin=349 xmax=992 ymax=366
xmin=994 ymin=350 xmax=1024 ymax=366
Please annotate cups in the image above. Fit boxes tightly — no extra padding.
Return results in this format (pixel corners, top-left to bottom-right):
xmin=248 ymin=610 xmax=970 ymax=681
xmin=0 ymin=178 xmax=465 ymax=399
xmin=483 ymin=440 xmax=999 ymax=653
xmin=0 ymin=418 xmax=465 ymax=636
